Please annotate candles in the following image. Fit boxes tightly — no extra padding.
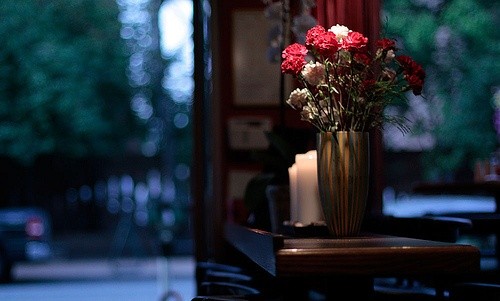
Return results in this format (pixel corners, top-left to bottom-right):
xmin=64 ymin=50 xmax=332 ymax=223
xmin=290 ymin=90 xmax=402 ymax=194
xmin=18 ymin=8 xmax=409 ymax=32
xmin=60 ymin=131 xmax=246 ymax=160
xmin=288 ymin=163 xmax=298 ymax=227
xmin=296 ymin=150 xmax=323 ymax=226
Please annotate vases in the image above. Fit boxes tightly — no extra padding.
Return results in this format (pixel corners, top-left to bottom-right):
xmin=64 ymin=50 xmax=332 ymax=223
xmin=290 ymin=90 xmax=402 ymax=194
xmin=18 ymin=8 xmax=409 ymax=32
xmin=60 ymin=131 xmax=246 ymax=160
xmin=317 ymin=132 xmax=382 ymax=239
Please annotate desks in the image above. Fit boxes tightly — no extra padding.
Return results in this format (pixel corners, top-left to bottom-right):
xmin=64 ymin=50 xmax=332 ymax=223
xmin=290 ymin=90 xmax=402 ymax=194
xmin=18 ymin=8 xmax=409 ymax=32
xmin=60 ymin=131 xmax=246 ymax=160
xmin=220 ymin=230 xmax=480 ymax=301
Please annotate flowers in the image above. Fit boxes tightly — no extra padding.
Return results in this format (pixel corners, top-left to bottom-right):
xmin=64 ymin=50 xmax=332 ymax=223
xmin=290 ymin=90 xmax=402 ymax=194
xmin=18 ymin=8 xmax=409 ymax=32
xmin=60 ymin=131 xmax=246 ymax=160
xmin=281 ymin=17 xmax=425 ymax=137
